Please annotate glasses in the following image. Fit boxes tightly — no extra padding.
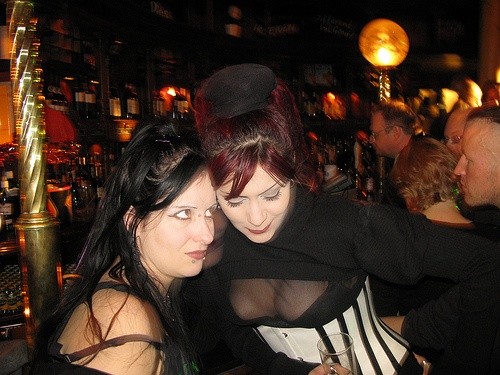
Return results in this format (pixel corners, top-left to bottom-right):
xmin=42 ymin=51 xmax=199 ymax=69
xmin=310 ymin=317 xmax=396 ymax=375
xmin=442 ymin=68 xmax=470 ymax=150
xmin=370 ymin=124 xmax=403 ymax=139
xmin=395 ymin=180 xmax=409 ymax=201
xmin=440 ymin=134 xmax=462 ymax=145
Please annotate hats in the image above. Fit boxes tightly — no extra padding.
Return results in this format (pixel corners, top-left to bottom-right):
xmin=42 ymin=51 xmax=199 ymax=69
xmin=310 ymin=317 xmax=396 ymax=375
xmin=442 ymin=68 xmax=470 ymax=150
xmin=206 ymin=63 xmax=278 ymax=117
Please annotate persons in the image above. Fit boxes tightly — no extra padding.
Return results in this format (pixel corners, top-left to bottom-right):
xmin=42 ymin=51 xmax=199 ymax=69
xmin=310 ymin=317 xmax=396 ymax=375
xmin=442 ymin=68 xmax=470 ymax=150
xmin=324 ymin=78 xmax=499 ymax=374
xmin=181 ymin=63 xmax=500 ymax=375
xmin=31 ymin=120 xmax=216 ymax=375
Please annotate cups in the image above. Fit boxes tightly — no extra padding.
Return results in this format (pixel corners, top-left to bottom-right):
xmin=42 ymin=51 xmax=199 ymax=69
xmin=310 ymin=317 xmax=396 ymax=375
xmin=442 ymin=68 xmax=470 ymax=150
xmin=318 ymin=333 xmax=357 ymax=375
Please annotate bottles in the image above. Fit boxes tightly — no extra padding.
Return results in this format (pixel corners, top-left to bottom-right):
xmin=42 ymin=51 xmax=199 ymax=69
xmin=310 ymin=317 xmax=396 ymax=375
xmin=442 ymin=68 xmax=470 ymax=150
xmin=40 ymin=72 xmax=190 ymax=225
xmin=299 ymin=89 xmax=384 ymax=201
xmin=0 ymin=158 xmax=20 ymax=241
